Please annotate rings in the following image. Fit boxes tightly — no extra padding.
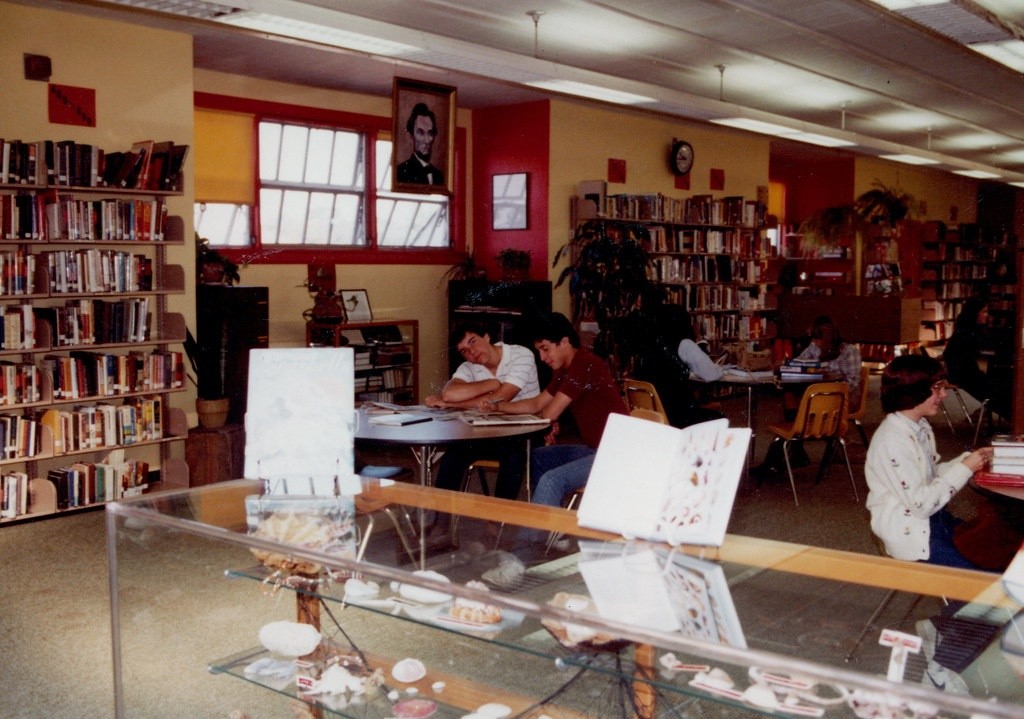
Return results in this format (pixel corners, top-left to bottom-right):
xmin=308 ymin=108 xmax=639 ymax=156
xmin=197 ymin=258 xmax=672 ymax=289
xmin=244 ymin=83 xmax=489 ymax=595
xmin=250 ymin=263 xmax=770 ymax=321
xmin=985 ymin=459 xmax=987 ymax=463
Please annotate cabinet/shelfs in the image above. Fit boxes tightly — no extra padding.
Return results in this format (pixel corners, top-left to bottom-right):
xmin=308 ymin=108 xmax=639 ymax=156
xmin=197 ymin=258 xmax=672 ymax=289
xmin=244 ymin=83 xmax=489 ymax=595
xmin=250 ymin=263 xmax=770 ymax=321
xmin=447 ymin=279 xmax=552 ymax=391
xmin=107 ymin=475 xmax=1024 ymax=719
xmin=570 ymin=197 xmax=777 ymax=400
xmin=306 ymin=319 xmax=420 ymax=481
xmin=921 ymin=221 xmax=1018 ymax=358
xmin=0 ymin=141 xmax=190 ymax=524
xmin=778 ymin=223 xmax=901 ymax=374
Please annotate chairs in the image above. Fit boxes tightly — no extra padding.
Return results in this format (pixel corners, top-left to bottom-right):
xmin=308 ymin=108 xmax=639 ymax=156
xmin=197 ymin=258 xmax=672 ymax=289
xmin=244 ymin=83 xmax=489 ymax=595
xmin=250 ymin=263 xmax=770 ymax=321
xmin=356 ymin=367 xmax=949 ymax=663
xmin=344 ymin=341 xmax=1024 ymax=664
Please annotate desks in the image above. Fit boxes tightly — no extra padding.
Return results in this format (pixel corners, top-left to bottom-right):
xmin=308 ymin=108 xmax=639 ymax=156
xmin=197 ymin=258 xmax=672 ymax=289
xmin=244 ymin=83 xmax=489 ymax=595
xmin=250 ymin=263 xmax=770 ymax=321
xmin=355 ymin=404 xmax=553 ymax=550
xmin=969 ymin=446 xmax=1024 ymax=500
xmin=689 ymin=364 xmax=848 ymax=461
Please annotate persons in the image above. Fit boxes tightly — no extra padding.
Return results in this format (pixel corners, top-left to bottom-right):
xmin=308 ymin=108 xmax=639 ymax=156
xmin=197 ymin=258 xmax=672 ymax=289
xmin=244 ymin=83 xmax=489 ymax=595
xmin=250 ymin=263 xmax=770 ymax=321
xmin=425 ymin=323 xmax=540 ymax=538
xmin=477 ymin=312 xmax=632 ymax=565
xmin=865 ymin=353 xmax=1000 ymax=691
xmin=627 ymin=299 xmax=1015 ymax=474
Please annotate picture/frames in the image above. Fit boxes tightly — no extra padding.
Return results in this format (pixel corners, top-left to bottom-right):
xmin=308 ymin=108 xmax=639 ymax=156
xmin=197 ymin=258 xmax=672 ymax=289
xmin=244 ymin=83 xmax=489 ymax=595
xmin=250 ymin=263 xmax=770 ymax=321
xmin=392 ymin=76 xmax=528 ymax=230
xmin=340 ymin=288 xmax=373 ymax=320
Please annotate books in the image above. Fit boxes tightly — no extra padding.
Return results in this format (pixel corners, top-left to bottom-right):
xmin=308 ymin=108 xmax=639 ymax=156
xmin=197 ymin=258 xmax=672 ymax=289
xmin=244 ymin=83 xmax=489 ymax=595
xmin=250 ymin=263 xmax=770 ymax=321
xmin=575 ymin=180 xmax=797 ymax=360
xmin=0 ymin=138 xmax=189 ymax=519
xmin=976 ymin=440 xmax=1024 ymax=487
xmin=917 ymin=224 xmax=995 ymax=343
xmin=344 ymin=344 xmax=433 ymax=426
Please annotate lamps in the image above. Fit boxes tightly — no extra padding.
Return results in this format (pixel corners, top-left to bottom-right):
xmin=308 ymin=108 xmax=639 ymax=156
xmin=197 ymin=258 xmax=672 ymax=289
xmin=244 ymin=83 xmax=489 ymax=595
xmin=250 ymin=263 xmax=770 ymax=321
xmin=244 ymin=348 xmax=355 ymax=512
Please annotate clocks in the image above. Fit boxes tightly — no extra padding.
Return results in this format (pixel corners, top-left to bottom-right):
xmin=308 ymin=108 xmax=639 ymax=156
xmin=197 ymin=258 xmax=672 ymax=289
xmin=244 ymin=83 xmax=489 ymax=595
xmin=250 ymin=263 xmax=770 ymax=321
xmin=671 ymin=141 xmax=695 ymax=176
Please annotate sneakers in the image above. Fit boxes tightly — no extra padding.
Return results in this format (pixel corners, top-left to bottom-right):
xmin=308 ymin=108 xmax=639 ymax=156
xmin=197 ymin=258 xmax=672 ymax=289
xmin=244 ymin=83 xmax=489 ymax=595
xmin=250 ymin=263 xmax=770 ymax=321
xmin=914 ymin=619 xmax=974 ymax=698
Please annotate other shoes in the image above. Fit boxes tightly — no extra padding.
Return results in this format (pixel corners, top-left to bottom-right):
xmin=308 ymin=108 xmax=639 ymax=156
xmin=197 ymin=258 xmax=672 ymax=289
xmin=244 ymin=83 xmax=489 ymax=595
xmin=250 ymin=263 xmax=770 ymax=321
xmin=546 ymin=529 xmax=571 ymax=551
xmin=480 ymin=552 xmax=525 ymax=591
xmin=788 ymin=439 xmax=811 ymax=467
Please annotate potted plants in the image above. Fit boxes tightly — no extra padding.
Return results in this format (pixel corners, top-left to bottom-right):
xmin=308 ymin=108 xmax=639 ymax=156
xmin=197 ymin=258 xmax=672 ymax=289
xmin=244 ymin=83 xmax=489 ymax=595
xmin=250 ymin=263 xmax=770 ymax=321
xmin=195 ymin=232 xmax=241 ymax=429
xmin=797 ymin=197 xmax=854 ymax=258
xmin=853 ymin=174 xmax=918 ymax=264
xmin=436 ymin=243 xmax=532 ymax=288
